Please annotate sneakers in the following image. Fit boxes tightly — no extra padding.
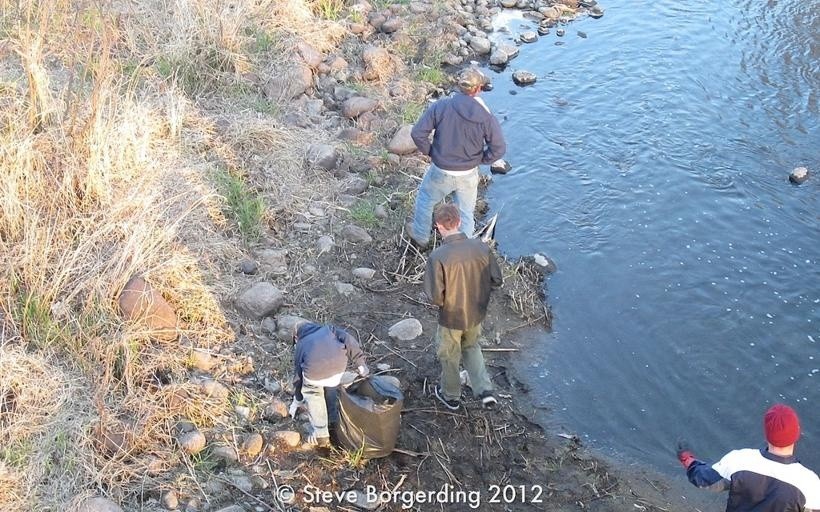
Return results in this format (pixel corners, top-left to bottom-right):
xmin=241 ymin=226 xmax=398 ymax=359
xmin=406 ymin=222 xmax=429 ymax=248
xmin=302 ymin=434 xmax=329 ymax=448
xmin=435 ymin=385 xmax=459 ymax=410
xmin=478 ymin=390 xmax=497 ymax=409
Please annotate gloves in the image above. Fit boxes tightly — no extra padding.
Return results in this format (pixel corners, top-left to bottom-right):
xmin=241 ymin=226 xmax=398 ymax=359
xmin=357 ymin=362 xmax=369 ymax=377
xmin=675 ymin=440 xmax=697 ymax=470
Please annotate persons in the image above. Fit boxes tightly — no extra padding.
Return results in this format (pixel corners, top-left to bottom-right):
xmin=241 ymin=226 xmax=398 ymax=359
xmin=292 ymin=318 xmax=371 ymax=447
xmin=675 ymin=403 xmax=820 ymax=512
xmin=422 ymin=202 xmax=504 ymax=411
xmin=405 ymin=65 xmax=508 ymax=252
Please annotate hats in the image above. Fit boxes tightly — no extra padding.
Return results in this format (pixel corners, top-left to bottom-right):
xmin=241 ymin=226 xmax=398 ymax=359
xmin=459 ymin=69 xmax=484 ymax=90
xmin=765 ymin=405 xmax=800 ymax=446
xmin=293 ymin=320 xmax=310 ymax=346
